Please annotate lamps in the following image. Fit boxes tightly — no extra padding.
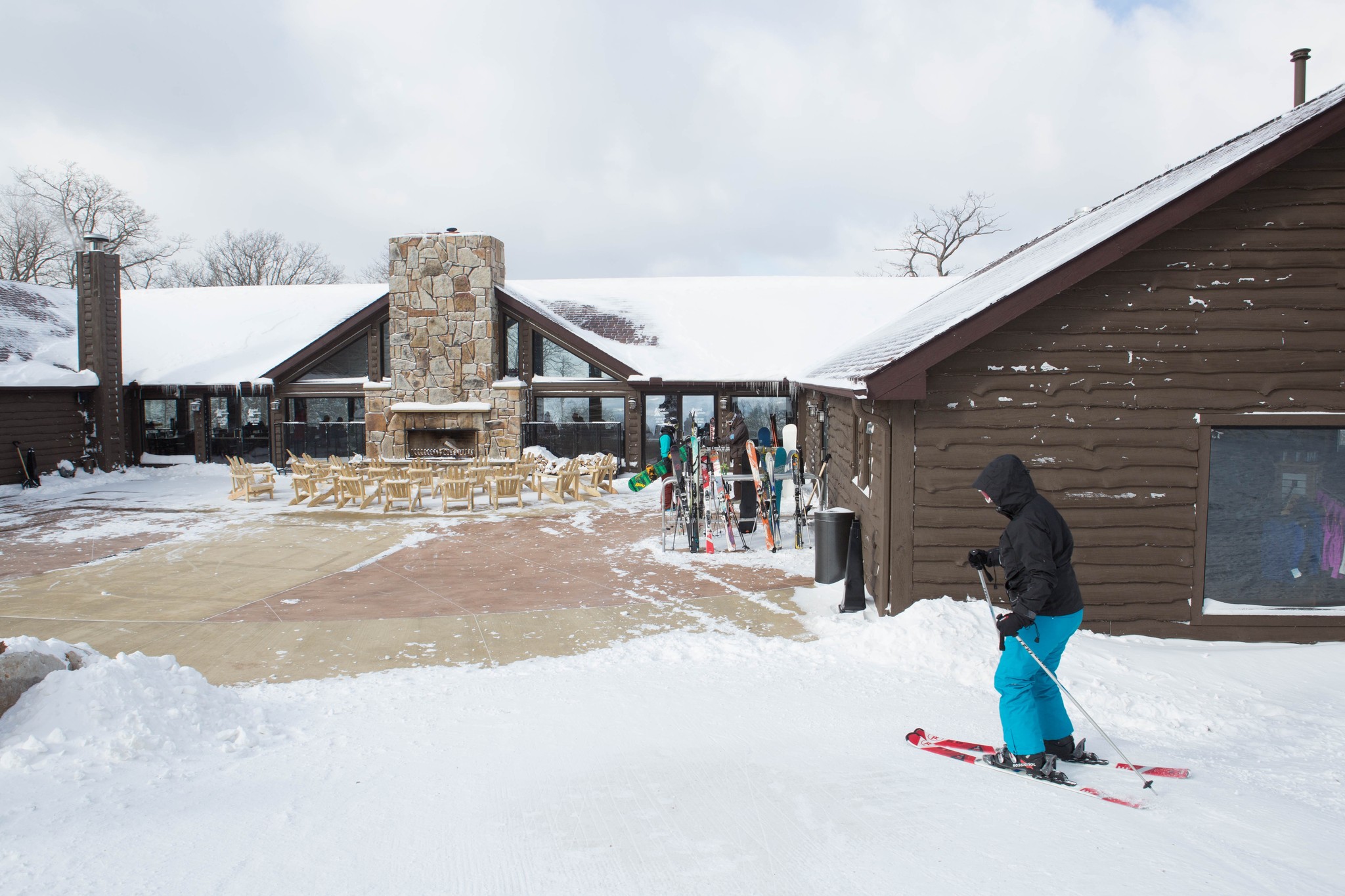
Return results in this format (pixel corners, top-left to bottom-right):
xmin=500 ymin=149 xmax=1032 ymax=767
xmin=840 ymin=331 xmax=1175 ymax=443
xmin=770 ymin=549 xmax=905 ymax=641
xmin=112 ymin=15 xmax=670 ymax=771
xmin=720 ymin=398 xmax=728 ymax=409
xmin=628 ymin=399 xmax=636 ymax=409
xmin=190 ymin=400 xmax=200 ymax=411
xmin=808 ymin=403 xmax=818 ymax=416
xmin=271 ymin=400 xmax=280 ymax=410
xmin=816 ymin=408 xmax=828 ymax=423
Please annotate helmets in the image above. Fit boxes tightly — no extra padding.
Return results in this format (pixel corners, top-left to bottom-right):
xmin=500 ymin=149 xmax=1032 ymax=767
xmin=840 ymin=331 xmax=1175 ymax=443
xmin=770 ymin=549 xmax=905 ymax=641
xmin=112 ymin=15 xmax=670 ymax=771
xmin=664 ymin=416 xmax=678 ymax=433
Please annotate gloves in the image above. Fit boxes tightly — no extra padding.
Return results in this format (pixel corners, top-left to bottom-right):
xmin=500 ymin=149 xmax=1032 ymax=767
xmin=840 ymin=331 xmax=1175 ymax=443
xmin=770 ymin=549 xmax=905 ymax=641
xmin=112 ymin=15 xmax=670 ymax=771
xmin=995 ymin=613 xmax=1026 ymax=637
xmin=968 ymin=548 xmax=989 ymax=567
xmin=706 ymin=440 xmax=718 ymax=447
xmin=663 ymin=457 xmax=672 ymax=473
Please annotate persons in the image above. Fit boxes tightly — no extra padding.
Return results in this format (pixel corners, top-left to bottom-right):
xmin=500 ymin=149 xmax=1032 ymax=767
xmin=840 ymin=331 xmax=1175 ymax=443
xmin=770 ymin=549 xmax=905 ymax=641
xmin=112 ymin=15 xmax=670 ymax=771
xmin=657 ymin=417 xmax=679 ymax=511
xmin=329 ymin=417 xmax=344 ymax=439
xmin=703 ymin=412 xmax=749 ymax=504
xmin=572 ymin=413 xmax=589 ymax=434
xmin=539 ymin=411 xmax=559 ymax=436
xmin=319 ymin=415 xmax=330 ymax=433
xmin=967 ymin=454 xmax=1076 ymax=774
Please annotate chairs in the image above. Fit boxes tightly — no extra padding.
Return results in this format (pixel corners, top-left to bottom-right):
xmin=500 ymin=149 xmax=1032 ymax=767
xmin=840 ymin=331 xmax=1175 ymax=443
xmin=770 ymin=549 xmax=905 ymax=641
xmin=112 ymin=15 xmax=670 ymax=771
xmin=146 ymin=427 xmax=278 ymax=502
xmin=287 ymin=428 xmax=619 ymax=513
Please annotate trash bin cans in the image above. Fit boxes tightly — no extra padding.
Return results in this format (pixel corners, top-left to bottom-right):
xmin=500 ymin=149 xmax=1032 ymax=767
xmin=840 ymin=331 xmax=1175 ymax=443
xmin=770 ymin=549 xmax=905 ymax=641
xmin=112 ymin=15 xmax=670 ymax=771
xmin=814 ymin=506 xmax=856 ymax=584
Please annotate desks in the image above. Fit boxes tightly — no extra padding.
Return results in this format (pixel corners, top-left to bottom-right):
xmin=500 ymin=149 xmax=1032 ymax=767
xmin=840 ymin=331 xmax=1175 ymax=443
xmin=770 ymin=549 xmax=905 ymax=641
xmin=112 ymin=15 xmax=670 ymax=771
xmin=152 ymin=434 xmax=185 ymax=454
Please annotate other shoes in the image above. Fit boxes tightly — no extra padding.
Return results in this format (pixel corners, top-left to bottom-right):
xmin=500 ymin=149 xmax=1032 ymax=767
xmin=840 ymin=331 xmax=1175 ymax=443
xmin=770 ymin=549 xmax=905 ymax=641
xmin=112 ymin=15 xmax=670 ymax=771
xmin=994 ymin=745 xmax=1046 ymax=775
xmin=730 ymin=497 xmax=741 ymax=504
xmin=1042 ymin=734 xmax=1074 ymax=759
xmin=666 ymin=506 xmax=675 ymax=511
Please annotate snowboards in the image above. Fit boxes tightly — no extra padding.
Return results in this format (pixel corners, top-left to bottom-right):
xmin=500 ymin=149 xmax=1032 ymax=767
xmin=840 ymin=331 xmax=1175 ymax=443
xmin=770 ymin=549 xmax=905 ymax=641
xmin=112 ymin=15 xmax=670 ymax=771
xmin=770 ymin=447 xmax=782 ymax=538
xmin=757 ymin=427 xmax=772 ymax=490
xmin=738 ymin=447 xmax=761 ymax=534
xmin=782 ymin=423 xmax=797 ymax=499
xmin=627 ymin=438 xmax=689 ymax=493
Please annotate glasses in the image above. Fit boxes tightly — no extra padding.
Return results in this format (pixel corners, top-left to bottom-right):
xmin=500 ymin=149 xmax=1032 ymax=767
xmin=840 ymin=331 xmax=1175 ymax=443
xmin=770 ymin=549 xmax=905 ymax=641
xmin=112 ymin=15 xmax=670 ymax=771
xmin=978 ymin=489 xmax=991 ymax=505
xmin=674 ymin=423 xmax=679 ymax=429
xmin=726 ymin=421 xmax=733 ymax=425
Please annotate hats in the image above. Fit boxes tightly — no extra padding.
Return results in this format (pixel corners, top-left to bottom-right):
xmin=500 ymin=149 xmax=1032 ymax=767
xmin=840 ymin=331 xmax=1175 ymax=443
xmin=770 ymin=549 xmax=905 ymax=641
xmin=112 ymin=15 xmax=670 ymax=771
xmin=726 ymin=412 xmax=737 ymax=428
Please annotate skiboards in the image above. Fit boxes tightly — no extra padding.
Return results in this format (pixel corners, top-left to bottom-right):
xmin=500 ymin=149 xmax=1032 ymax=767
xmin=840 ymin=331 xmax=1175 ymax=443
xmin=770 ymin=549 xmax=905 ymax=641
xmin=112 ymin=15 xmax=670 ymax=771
xmin=769 ymin=413 xmax=779 ymax=448
xmin=906 ymin=727 xmax=1191 ymax=810
xmin=689 ymin=411 xmax=699 ymax=439
xmin=671 ymin=434 xmax=806 ymax=553
xmin=709 ymin=417 xmax=716 ymax=451
